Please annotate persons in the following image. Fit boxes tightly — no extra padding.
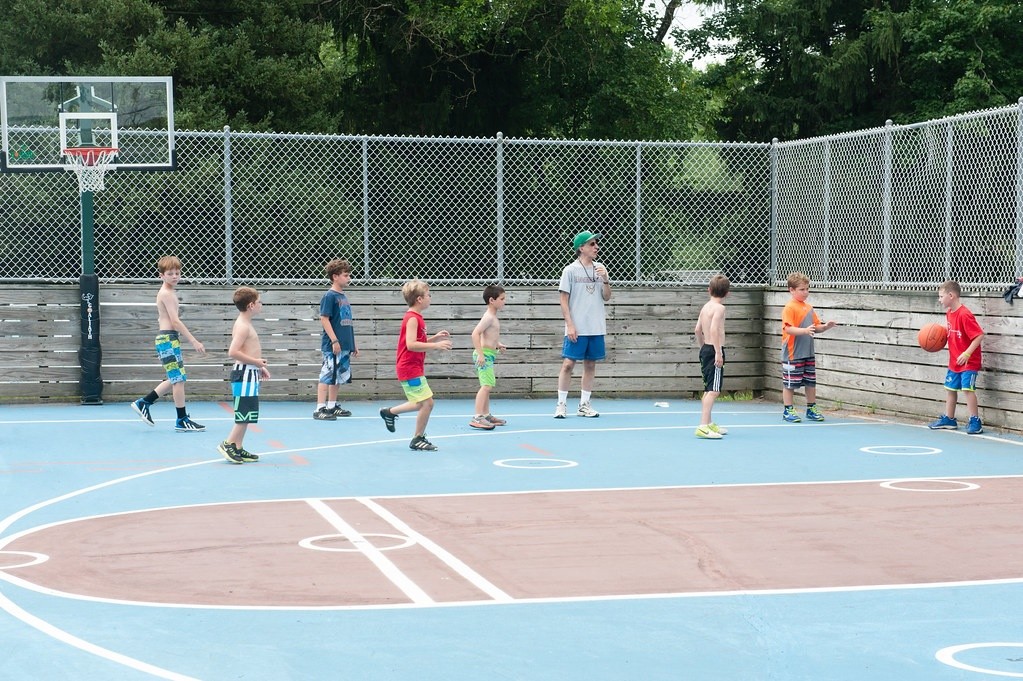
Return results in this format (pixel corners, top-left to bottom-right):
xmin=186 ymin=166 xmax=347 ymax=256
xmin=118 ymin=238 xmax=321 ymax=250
xmin=380 ymin=281 xmax=453 ymax=451
xmin=782 ymin=272 xmax=837 ymax=423
xmin=695 ymin=275 xmax=730 ymax=438
xmin=217 ymin=288 xmax=270 ymax=463
xmin=929 ymin=281 xmax=984 ymax=433
xmin=130 ymin=257 xmax=206 ymax=431
xmin=469 ymin=286 xmax=506 ymax=430
xmin=313 ymin=260 xmax=359 ymax=421
xmin=555 ymin=231 xmax=611 ymax=418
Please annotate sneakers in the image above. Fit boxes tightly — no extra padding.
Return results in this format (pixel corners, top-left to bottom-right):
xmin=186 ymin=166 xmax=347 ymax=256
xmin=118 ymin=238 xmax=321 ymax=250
xmin=326 ymin=403 xmax=352 ymax=417
xmin=553 ymin=401 xmax=569 ymax=418
xmin=216 ymin=440 xmax=244 ymax=464
xmin=927 ymin=414 xmax=959 ymax=430
xmin=312 ymin=406 xmax=337 ymax=421
xmin=469 ymin=415 xmax=496 ymax=430
xmin=694 ymin=425 xmax=723 ymax=439
xmin=484 ymin=413 xmax=507 ymax=426
xmin=708 ymin=422 xmax=728 ymax=435
xmin=965 ymin=416 xmax=984 ymax=434
xmin=409 ymin=433 xmax=438 ymax=451
xmin=175 ymin=414 xmax=206 ymax=432
xmin=805 ymin=405 xmax=825 ymax=421
xmin=235 ymin=447 xmax=259 ymax=462
xmin=130 ymin=397 xmax=155 ymax=427
xmin=577 ymin=402 xmax=600 ymax=418
xmin=380 ymin=406 xmax=399 ymax=432
xmin=782 ymin=408 xmax=802 ymax=423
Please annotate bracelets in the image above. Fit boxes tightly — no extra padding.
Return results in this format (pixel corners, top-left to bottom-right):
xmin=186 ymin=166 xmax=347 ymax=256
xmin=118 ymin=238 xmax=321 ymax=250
xmin=603 ymin=278 xmax=610 ymax=284
xmin=963 ymin=352 xmax=970 ymax=357
xmin=331 ymin=339 xmax=339 ymax=345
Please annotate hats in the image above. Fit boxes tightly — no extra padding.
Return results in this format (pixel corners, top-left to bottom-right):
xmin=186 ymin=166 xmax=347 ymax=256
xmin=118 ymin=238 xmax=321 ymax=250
xmin=573 ymin=231 xmax=601 ymax=250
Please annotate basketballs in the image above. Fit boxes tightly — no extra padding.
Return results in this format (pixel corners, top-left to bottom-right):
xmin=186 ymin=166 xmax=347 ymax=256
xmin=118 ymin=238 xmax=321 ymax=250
xmin=918 ymin=323 xmax=947 ymax=352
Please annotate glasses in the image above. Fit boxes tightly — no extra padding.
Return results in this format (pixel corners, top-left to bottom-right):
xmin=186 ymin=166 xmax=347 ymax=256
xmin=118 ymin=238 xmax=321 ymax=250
xmin=584 ymin=241 xmax=598 ymax=246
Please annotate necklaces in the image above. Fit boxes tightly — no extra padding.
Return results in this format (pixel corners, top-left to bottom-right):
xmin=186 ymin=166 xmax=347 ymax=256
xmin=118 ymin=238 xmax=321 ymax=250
xmin=578 ymin=258 xmax=599 ymax=282
